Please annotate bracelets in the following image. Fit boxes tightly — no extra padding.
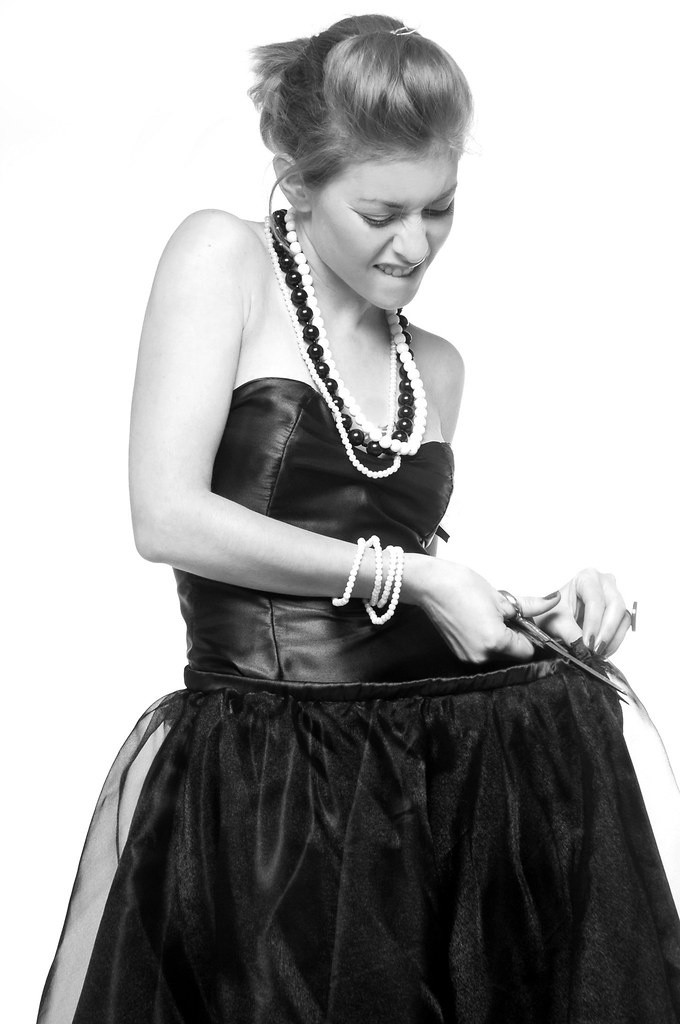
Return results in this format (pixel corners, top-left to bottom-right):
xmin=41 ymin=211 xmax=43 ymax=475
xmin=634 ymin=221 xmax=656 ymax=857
xmin=333 ymin=535 xmax=404 ymax=624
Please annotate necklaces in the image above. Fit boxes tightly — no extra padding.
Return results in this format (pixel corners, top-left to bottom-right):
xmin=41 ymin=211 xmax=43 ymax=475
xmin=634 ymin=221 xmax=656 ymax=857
xmin=265 ymin=208 xmax=428 ymax=478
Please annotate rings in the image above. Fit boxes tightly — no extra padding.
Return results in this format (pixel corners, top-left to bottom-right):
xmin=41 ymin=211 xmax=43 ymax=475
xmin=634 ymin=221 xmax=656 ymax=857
xmin=625 ymin=602 xmax=637 ymax=631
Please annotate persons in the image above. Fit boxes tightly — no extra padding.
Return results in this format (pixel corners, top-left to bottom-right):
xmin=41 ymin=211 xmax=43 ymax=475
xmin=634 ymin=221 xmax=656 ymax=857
xmin=38 ymin=14 xmax=680 ymax=1023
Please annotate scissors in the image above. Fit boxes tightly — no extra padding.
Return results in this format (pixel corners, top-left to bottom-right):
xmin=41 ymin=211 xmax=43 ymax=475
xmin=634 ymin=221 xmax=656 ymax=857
xmin=497 ymin=586 xmax=635 ymax=711
